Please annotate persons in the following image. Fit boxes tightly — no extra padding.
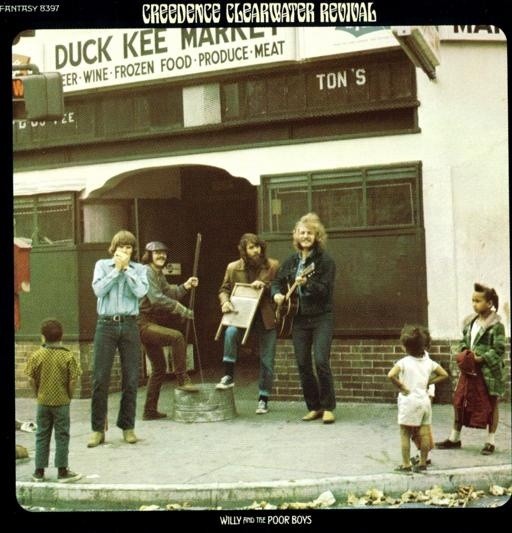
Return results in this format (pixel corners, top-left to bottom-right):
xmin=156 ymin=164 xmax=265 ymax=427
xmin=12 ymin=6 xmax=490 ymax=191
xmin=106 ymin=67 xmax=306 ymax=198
xmin=270 ymin=213 xmax=336 ymax=424
xmin=24 ymin=320 xmax=82 ymax=483
xmin=410 ymin=326 xmax=436 ymax=466
xmin=87 ymin=230 xmax=150 ymax=448
xmin=215 ymin=233 xmax=282 ymax=414
xmin=387 ymin=324 xmax=448 ymax=473
xmin=137 ymin=240 xmax=199 ymax=421
xmin=435 ymin=283 xmax=505 ymax=455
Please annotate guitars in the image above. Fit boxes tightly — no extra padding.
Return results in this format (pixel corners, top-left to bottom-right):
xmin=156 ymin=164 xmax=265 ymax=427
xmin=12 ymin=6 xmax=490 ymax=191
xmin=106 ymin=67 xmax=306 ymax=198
xmin=273 ymin=262 xmax=320 ymax=338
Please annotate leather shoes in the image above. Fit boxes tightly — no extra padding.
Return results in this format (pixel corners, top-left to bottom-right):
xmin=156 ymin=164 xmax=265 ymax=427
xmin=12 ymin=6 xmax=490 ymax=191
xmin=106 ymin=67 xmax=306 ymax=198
xmin=481 ymin=443 xmax=495 ymax=454
xmin=87 ymin=430 xmax=106 ymax=448
xmin=434 ymin=438 xmax=461 ymax=449
xmin=120 ymin=430 xmax=137 ymax=444
xmin=323 ymin=409 xmax=336 ymax=424
xmin=302 ymin=408 xmax=322 ymax=421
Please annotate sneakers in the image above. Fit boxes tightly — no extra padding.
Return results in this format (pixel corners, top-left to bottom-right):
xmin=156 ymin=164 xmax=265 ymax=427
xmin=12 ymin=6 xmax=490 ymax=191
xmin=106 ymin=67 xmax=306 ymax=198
xmin=215 ymin=376 xmax=234 ymax=390
xmin=58 ymin=470 xmax=84 ymax=484
xmin=143 ymin=408 xmax=169 ymax=420
xmin=175 ymin=376 xmax=199 ymax=393
xmin=255 ymin=399 xmax=269 ymax=415
xmin=411 ymin=454 xmax=431 ymax=466
xmin=32 ymin=472 xmax=44 ymax=483
xmin=412 ymin=463 xmax=427 ymax=474
xmin=393 ymin=464 xmax=412 ymax=474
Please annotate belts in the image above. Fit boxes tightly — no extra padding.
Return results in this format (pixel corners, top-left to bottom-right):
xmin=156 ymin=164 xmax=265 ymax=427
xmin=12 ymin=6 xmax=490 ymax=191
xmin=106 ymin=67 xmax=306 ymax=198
xmin=97 ymin=314 xmax=138 ymax=323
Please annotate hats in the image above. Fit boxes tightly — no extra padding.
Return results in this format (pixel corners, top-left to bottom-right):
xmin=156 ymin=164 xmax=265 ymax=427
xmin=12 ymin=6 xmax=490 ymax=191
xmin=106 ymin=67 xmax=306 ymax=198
xmin=144 ymin=240 xmax=169 ymax=253
